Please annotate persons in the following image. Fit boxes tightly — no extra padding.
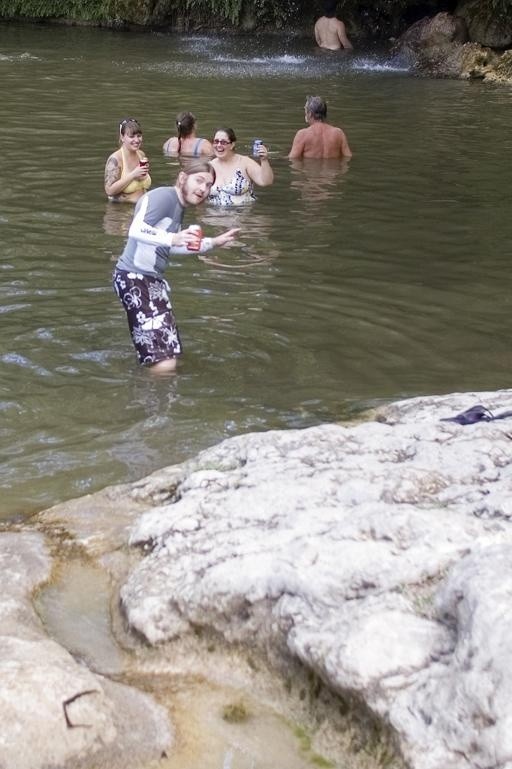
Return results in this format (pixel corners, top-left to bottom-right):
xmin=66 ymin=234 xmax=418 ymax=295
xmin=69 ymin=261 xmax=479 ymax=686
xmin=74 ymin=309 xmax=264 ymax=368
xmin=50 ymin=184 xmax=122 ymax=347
xmin=112 ymin=160 xmax=241 ymax=371
xmin=314 ymin=1 xmax=353 ymax=50
xmin=104 ymin=118 xmax=152 ymax=204
xmin=203 ymin=127 xmax=274 ymax=207
xmin=163 ymin=112 xmax=215 ymax=159
xmin=288 ymin=96 xmax=352 ymax=160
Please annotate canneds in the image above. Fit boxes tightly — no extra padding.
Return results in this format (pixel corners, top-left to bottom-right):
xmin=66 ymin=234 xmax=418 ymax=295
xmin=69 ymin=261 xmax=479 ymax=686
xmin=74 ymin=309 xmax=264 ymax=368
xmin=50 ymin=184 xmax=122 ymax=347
xmin=187 ymin=224 xmax=202 ymax=251
xmin=139 ymin=157 xmax=149 ymax=175
xmin=253 ymin=139 xmax=263 ymax=157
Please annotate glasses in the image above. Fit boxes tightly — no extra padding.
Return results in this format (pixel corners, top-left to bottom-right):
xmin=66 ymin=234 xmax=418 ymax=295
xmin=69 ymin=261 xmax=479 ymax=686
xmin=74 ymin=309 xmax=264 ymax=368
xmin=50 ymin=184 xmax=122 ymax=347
xmin=119 ymin=119 xmax=139 ymax=139
xmin=211 ymin=138 xmax=232 ymax=146
